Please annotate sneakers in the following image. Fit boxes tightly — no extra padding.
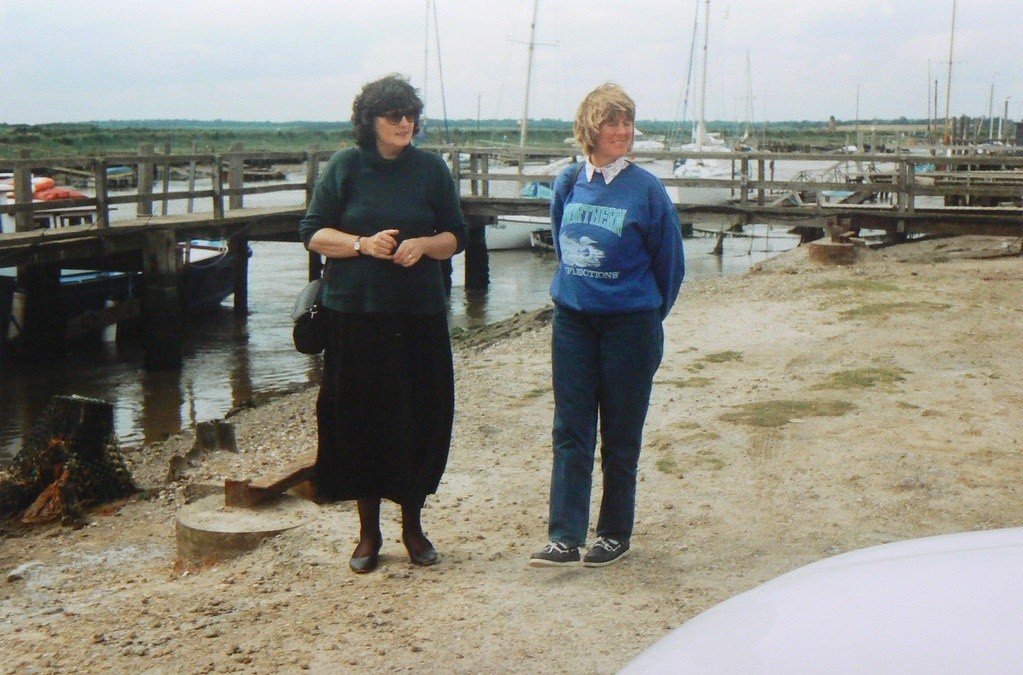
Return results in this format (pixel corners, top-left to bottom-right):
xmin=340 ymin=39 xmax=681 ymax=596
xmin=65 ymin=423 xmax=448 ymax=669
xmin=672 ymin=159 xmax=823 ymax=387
xmin=529 ymin=538 xmax=580 ymax=569
xmin=583 ymin=536 xmax=630 ymax=568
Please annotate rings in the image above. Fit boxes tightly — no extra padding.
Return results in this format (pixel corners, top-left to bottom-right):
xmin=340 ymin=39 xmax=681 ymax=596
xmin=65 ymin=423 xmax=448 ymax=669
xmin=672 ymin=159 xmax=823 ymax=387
xmin=409 ymin=254 xmax=413 ymax=260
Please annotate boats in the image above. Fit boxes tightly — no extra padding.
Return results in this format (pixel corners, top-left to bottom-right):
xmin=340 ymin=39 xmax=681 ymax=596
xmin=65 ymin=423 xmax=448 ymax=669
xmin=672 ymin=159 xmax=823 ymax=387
xmin=0 ymin=171 xmax=253 ymax=358
xmin=725 ymin=185 xmax=878 ymax=207
xmin=89 ymin=167 xmax=136 ymax=191
xmin=220 ymin=162 xmax=288 ymax=183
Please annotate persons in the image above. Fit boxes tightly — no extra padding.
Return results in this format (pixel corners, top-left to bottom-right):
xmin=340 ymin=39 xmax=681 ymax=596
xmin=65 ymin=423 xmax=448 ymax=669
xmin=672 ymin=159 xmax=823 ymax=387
xmin=298 ymin=77 xmax=470 ymax=573
xmin=529 ymin=82 xmax=687 ymax=567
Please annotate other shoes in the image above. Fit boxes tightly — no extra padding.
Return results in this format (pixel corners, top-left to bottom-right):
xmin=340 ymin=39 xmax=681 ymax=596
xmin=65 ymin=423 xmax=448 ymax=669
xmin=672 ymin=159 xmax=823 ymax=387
xmin=350 ymin=533 xmax=382 ymax=573
xmin=401 ymin=531 xmax=436 ymax=566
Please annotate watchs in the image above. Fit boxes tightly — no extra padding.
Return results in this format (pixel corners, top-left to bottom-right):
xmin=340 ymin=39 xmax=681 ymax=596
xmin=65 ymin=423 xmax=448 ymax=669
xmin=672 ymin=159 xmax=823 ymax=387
xmin=354 ymin=236 xmax=365 ymax=256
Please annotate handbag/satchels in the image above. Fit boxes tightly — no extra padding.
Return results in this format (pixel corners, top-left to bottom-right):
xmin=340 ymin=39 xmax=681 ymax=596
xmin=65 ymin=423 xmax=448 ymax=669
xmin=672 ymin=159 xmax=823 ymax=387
xmin=293 ymin=278 xmax=326 ymax=355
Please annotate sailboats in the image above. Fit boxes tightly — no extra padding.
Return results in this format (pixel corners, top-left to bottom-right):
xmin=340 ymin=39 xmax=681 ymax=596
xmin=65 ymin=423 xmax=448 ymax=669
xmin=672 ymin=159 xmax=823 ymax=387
xmin=416 ymin=0 xmax=768 ymax=252
xmin=825 ymin=0 xmax=1015 ymax=184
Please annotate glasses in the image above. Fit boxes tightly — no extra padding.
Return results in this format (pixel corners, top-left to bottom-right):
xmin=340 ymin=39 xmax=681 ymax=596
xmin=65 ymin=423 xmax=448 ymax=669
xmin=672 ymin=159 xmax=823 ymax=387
xmin=374 ymin=111 xmax=420 ymax=125
